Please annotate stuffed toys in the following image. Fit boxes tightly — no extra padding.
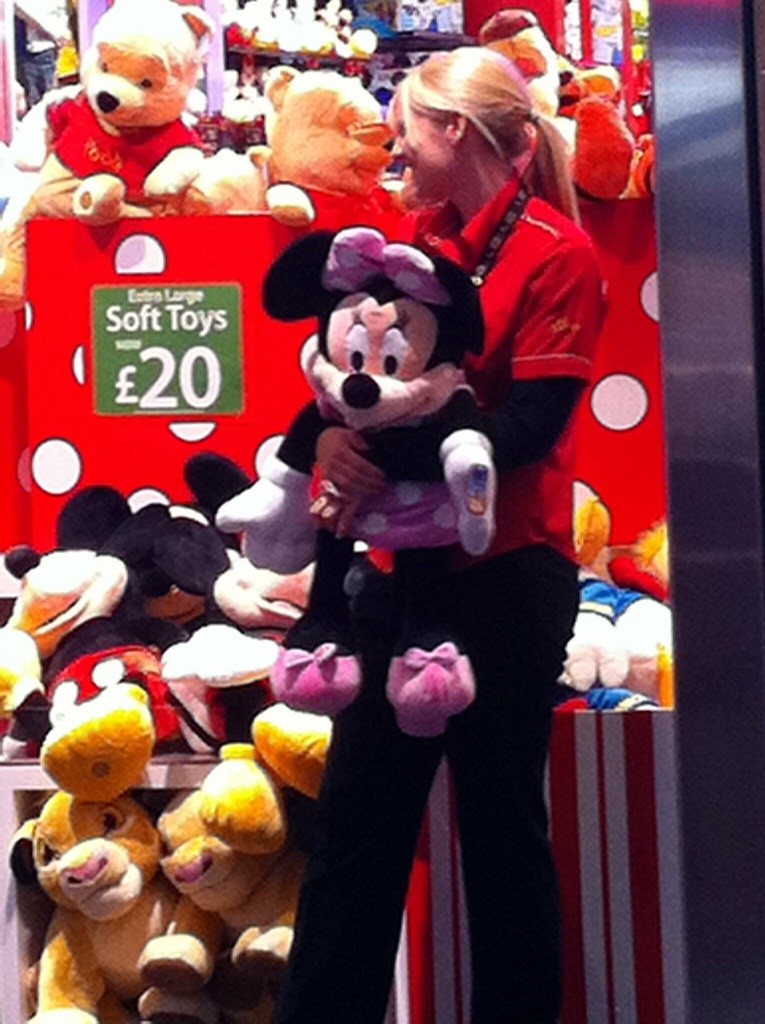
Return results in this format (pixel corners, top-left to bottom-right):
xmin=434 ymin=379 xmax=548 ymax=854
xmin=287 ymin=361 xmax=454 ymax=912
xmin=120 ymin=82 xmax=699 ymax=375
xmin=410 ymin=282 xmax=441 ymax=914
xmin=217 ymin=225 xmax=495 ymax=736
xmin=555 ymin=478 xmax=673 ymax=710
xmin=1 ymin=450 xmax=334 ymax=1023
xmin=0 ymin=0 xmax=656 ymax=350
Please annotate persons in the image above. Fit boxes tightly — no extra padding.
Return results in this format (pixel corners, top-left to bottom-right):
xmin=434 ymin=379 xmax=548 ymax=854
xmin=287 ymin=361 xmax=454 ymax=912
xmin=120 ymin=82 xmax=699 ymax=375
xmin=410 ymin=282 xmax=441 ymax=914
xmin=270 ymin=49 xmax=610 ymax=1024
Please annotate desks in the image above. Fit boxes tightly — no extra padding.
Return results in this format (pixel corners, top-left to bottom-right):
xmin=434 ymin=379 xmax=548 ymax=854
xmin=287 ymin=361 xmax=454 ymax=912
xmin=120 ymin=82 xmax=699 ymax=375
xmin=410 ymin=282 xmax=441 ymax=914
xmin=0 ymin=706 xmax=675 ymax=1024
